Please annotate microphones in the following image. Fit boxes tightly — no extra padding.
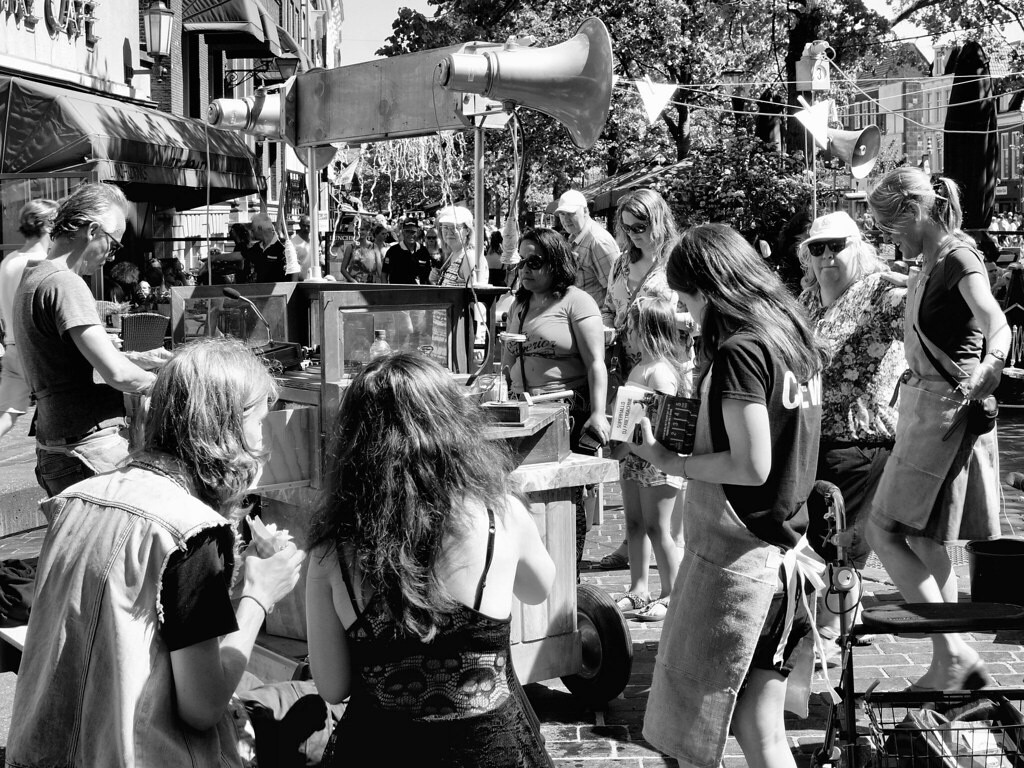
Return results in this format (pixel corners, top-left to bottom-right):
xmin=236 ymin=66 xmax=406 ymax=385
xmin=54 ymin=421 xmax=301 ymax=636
xmin=221 ymin=287 xmax=304 ymax=373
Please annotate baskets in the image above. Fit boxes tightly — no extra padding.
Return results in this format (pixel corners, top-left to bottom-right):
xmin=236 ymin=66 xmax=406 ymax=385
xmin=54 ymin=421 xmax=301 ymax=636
xmin=864 ymin=693 xmax=1024 ymax=768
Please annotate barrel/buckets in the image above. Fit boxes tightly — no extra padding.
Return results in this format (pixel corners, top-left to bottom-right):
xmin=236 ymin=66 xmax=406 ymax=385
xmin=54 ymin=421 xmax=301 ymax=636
xmin=966 ymin=538 xmax=1024 ymax=607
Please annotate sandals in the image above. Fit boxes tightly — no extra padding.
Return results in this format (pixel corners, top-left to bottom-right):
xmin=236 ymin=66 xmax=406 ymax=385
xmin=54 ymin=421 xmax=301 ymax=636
xmin=634 ymin=596 xmax=672 ymax=622
xmin=812 ymin=622 xmax=841 ymax=668
xmin=850 ymin=606 xmax=876 ymax=646
xmin=615 ymin=589 xmax=652 ymax=618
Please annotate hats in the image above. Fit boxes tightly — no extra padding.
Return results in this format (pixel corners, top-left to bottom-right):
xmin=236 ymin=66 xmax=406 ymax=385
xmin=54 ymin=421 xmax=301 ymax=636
xmin=439 ymin=206 xmax=474 ymax=230
xmin=402 ymin=224 xmax=419 ymax=232
xmin=799 ymin=211 xmax=861 ymax=250
xmin=552 ymin=187 xmax=587 ymax=214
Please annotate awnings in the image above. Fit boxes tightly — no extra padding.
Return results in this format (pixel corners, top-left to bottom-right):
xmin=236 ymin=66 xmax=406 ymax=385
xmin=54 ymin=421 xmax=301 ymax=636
xmin=183 ymin=0 xmax=314 ymax=79
xmin=2 ymin=77 xmax=260 ymax=210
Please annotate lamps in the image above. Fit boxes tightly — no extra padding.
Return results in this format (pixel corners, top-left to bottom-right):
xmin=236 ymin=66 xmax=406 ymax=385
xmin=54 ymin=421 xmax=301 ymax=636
xmin=226 ymin=47 xmax=300 ymax=93
xmin=123 ymin=0 xmax=174 ymax=84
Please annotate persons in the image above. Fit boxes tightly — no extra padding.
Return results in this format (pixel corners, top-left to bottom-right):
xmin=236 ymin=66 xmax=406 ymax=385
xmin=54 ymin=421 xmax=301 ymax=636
xmin=0 ymin=189 xmax=699 ymax=768
xmin=627 ymin=225 xmax=823 ymax=768
xmin=977 ymin=210 xmax=1024 ymax=302
xmin=793 ymin=211 xmax=910 ymax=668
xmin=867 ymin=168 xmax=1011 ymax=693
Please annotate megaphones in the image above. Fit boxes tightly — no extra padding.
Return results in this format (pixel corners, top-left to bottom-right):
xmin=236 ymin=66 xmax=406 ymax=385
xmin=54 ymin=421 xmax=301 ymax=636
xmin=815 ymin=124 xmax=881 ymax=179
xmin=438 ymin=15 xmax=613 ymax=151
xmin=208 ymin=67 xmax=338 ymax=170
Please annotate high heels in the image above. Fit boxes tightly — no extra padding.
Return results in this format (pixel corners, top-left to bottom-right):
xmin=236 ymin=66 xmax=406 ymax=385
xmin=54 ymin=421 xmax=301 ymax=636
xmin=901 ymin=658 xmax=991 ymax=705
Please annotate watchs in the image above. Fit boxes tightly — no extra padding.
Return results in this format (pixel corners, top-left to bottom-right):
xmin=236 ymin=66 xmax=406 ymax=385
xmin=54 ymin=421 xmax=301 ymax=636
xmin=984 ymin=349 xmax=1008 ymax=366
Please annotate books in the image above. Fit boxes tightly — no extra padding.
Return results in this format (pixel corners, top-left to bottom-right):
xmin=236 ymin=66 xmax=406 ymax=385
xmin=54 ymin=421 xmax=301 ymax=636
xmin=608 ymin=385 xmax=698 ymax=455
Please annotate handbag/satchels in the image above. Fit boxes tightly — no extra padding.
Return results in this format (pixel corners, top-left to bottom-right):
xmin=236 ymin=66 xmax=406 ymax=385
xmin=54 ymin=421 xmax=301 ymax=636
xmin=522 ymin=373 xmax=594 ymax=432
xmin=603 ymin=336 xmax=632 ymax=404
xmin=968 ymin=396 xmax=999 ymax=436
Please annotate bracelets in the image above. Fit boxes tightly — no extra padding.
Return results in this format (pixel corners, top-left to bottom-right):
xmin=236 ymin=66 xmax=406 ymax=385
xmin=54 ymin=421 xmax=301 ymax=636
xmin=681 ymin=457 xmax=694 ymax=482
xmin=240 ymin=596 xmax=268 ymax=618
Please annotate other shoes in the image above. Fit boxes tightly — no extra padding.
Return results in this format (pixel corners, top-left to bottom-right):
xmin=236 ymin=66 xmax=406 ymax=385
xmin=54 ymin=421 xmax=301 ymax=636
xmin=600 ymin=539 xmax=629 ymax=568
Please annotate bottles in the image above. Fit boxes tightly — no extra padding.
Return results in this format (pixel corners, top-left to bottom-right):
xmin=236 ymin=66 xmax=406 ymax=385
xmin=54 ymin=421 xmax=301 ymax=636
xmin=479 ymin=374 xmax=508 ymax=403
xmin=370 ymin=330 xmax=390 ymax=362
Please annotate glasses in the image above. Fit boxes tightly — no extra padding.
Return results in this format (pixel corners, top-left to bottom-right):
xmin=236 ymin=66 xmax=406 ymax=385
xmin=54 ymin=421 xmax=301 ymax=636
xmin=426 ymin=235 xmax=437 ymax=241
xmin=620 ymin=221 xmax=651 ymax=234
xmin=808 ymin=237 xmax=847 ymax=257
xmin=874 ymin=204 xmax=909 ymax=238
xmin=516 ymin=253 xmax=546 ymax=271
xmin=381 ymin=232 xmax=388 ymax=236
xmin=81 ymin=219 xmax=125 ymax=258
xmin=439 ymin=225 xmax=466 ymax=235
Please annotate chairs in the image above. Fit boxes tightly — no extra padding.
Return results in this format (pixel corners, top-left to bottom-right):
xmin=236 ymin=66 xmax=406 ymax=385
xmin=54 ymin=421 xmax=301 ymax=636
xmin=119 ymin=313 xmax=173 ymax=350
